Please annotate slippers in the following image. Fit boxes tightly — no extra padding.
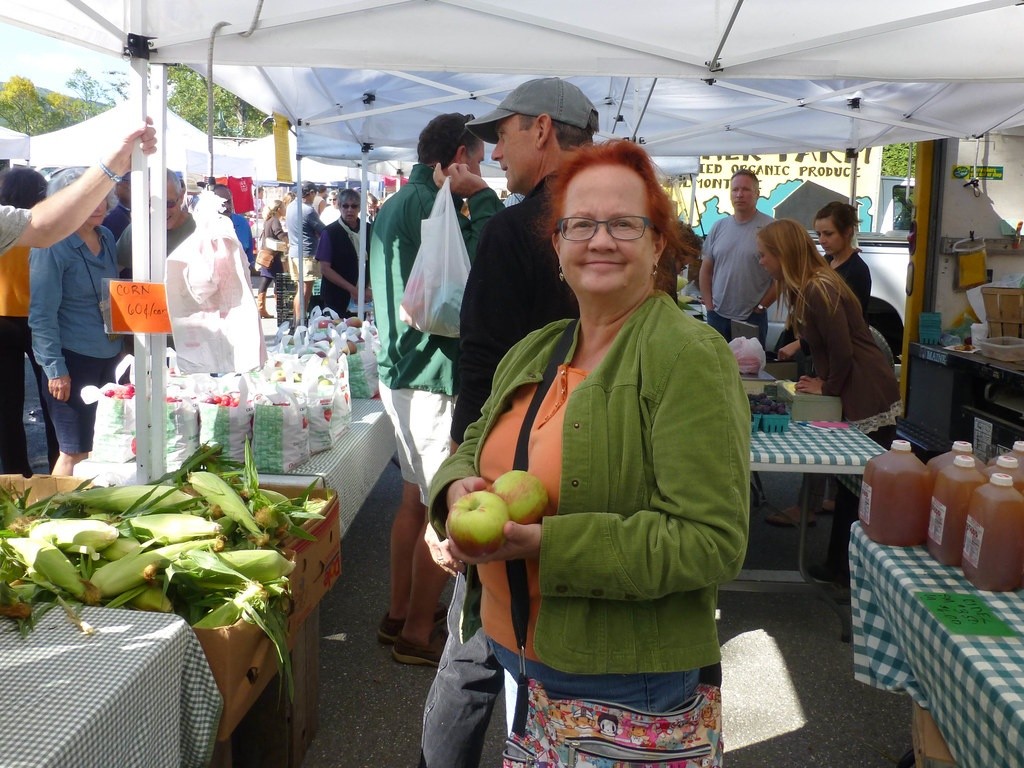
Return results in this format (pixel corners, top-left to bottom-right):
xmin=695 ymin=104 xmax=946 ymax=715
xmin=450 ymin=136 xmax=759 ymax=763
xmin=763 ymin=507 xmax=818 ymax=526
xmin=820 ymin=499 xmax=836 ymax=513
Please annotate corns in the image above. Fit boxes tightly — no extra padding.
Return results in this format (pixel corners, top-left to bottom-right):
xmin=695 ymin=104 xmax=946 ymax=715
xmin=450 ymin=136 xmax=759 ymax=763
xmin=0 ymin=447 xmax=296 ymax=614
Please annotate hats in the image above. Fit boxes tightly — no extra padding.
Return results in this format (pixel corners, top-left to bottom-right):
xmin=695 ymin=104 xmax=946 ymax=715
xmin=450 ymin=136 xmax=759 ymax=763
xmin=298 ymin=181 xmax=317 ymax=198
xmin=464 ymin=76 xmax=599 ymax=145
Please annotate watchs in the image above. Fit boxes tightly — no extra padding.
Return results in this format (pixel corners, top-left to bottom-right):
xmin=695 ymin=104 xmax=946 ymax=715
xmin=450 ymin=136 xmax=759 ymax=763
xmin=757 ymin=304 xmax=767 ymax=310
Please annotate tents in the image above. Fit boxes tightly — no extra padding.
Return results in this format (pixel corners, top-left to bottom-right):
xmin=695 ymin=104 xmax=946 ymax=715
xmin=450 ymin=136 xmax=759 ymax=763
xmin=0 ymin=0 xmax=1024 ymax=489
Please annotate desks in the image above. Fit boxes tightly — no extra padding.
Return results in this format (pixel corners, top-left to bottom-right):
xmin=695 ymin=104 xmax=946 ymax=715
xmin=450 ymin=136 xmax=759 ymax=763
xmin=288 ymin=394 xmax=400 ymax=541
xmin=0 ymin=604 xmax=224 ymax=767
xmin=710 ymin=414 xmax=888 ymax=589
xmin=848 ymin=517 xmax=1024 ymax=768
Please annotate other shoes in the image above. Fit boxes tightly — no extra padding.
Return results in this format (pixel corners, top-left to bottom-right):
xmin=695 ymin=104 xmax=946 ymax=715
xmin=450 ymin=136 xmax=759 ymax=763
xmin=808 ymin=564 xmax=844 ymax=583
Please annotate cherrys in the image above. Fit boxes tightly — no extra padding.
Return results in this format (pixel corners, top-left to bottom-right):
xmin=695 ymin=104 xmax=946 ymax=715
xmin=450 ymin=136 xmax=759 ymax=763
xmin=105 ymin=386 xmax=239 ymax=407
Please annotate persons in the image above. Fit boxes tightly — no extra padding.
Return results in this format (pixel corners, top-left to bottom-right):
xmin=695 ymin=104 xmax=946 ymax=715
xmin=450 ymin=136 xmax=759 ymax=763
xmin=253 ymin=188 xmax=266 ymax=212
xmin=367 ymin=192 xmax=380 ymax=224
xmin=111 ymin=165 xmax=197 ymax=350
xmin=767 ymin=201 xmax=904 ymax=588
xmin=191 ymin=182 xmax=207 ymax=210
xmin=0 ymin=116 xmax=158 ymax=474
xmin=213 ymin=183 xmax=254 ymax=264
xmin=256 ymin=201 xmax=289 ymax=320
xmin=756 ymin=218 xmax=902 ymax=452
xmin=319 ymin=190 xmax=342 ymax=226
xmin=652 ymin=217 xmax=700 ymax=301
xmin=283 ymin=192 xmax=297 ymax=203
xmin=699 ymin=171 xmax=785 ymax=354
xmin=423 ymin=137 xmax=752 ymax=767
xmin=450 ymin=76 xmax=600 ymax=455
xmin=29 ymin=165 xmax=119 ymax=474
xmin=313 ymin=184 xmax=328 ymax=215
xmin=314 ymin=189 xmax=371 ymax=319
xmin=370 ymin=112 xmax=507 ymax=659
xmin=288 ymin=180 xmax=327 ymax=332
xmin=103 ymin=170 xmax=131 ymax=245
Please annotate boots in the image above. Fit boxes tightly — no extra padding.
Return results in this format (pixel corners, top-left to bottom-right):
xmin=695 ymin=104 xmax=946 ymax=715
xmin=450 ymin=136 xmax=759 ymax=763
xmin=256 ymin=291 xmax=276 ymax=319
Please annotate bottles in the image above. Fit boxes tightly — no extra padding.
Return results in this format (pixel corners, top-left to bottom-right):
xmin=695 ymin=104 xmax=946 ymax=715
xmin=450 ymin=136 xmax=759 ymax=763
xmin=858 ymin=440 xmax=1024 ymax=591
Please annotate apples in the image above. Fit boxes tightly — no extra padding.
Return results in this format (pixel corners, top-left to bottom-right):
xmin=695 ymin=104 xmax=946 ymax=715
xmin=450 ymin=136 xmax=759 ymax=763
xmin=270 ymin=317 xmax=376 ymax=384
xmin=448 ymin=490 xmax=510 ymax=557
xmin=490 ymin=470 xmax=548 ymax=525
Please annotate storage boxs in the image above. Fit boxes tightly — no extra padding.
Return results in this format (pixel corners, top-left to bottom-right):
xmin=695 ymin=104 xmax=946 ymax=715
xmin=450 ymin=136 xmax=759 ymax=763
xmin=0 ymin=473 xmax=94 ymax=507
xmin=255 ymin=483 xmax=344 ymax=626
xmin=974 ymin=336 xmax=1023 ymax=361
xmin=190 ymin=601 xmax=296 ymax=745
xmin=776 ymin=380 xmax=842 ymax=421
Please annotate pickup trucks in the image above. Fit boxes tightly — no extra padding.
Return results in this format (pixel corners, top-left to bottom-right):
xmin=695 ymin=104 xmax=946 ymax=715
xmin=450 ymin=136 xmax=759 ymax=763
xmin=764 ymin=232 xmax=910 ymax=371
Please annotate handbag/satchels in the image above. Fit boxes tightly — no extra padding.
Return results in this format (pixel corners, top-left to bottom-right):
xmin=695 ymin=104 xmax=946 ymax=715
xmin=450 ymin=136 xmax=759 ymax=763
xmin=501 ymin=674 xmax=724 ymax=768
xmin=80 ymin=303 xmax=389 ymax=472
xmin=256 ymin=250 xmax=276 ymax=269
xmin=398 ymin=177 xmax=472 ymax=339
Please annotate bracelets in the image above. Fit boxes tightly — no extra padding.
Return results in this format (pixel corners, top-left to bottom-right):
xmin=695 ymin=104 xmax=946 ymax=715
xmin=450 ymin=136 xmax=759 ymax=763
xmin=99 ymin=162 xmax=122 ymax=183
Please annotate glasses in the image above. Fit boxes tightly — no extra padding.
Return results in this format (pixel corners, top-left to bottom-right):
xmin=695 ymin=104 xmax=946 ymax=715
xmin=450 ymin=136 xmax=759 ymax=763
xmin=167 ymin=195 xmax=181 ymax=208
xmin=342 ymin=204 xmax=360 ymax=209
xmin=553 ymin=217 xmax=664 ymax=243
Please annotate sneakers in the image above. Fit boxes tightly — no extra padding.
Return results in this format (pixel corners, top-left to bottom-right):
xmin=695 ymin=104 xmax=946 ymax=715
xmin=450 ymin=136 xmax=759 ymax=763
xmin=390 ymin=630 xmax=445 ymax=666
xmin=377 ymin=602 xmax=449 ymax=645
